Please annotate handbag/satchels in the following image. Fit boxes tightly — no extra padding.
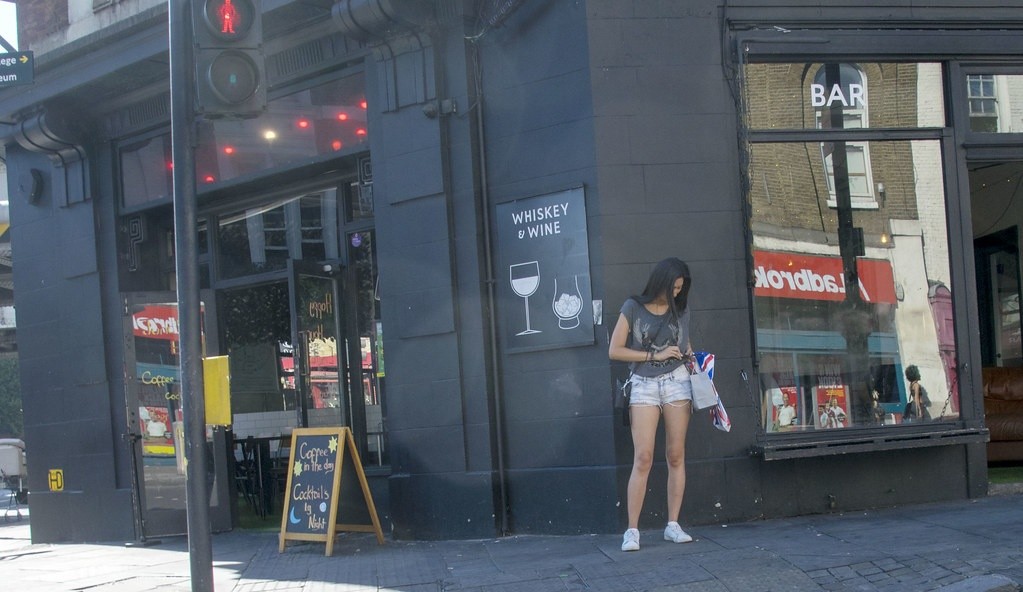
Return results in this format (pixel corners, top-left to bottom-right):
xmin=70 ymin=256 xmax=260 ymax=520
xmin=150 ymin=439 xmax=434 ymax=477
xmin=690 ymin=357 xmax=718 ymax=413
xmin=614 ymin=377 xmax=626 ymax=408
xmin=902 ymin=403 xmax=911 ymax=419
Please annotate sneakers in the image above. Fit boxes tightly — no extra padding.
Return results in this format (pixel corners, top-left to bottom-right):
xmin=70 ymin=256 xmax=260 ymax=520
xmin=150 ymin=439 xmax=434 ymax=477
xmin=664 ymin=524 xmax=692 ymax=542
xmin=622 ymin=530 xmax=640 ymax=550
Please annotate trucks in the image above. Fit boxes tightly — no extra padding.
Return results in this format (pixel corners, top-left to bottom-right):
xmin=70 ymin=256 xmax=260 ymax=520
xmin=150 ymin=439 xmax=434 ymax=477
xmin=4 ymin=453 xmax=29 ymax=504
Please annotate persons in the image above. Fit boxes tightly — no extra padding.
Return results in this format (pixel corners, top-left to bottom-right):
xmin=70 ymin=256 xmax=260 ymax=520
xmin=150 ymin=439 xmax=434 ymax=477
xmin=776 ymin=392 xmax=796 ymax=427
xmin=819 ymin=398 xmax=845 ymax=428
xmin=905 ymin=365 xmax=931 ymax=422
xmin=608 ymin=258 xmax=692 ymax=551
xmin=146 ymin=413 xmax=168 ymax=439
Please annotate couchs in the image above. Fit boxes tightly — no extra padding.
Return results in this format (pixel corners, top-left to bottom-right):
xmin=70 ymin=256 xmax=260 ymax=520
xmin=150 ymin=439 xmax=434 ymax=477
xmin=981 ymin=366 xmax=1023 ymax=468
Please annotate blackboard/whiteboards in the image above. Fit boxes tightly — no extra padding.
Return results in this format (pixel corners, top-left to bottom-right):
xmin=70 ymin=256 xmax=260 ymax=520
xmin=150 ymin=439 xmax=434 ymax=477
xmin=279 ymin=426 xmax=384 ymax=542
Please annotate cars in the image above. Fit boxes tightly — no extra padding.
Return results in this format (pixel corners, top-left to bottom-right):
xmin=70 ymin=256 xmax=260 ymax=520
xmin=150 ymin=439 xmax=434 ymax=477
xmin=145 ymin=466 xmax=187 ymax=508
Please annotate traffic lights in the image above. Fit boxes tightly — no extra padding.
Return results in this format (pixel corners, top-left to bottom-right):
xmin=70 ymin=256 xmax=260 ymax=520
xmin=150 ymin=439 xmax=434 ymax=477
xmin=190 ymin=0 xmax=265 ymax=122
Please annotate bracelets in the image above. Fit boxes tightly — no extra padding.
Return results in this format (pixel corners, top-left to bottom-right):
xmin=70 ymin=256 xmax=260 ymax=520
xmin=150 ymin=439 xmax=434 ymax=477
xmin=651 ymin=351 xmax=654 ymax=362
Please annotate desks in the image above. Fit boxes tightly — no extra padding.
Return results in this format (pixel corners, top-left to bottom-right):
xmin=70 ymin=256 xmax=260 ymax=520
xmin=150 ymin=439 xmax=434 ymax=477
xmin=234 ymin=434 xmax=293 ymax=514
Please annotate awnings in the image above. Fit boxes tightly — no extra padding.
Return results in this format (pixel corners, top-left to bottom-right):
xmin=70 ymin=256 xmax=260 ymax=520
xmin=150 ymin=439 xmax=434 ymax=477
xmin=281 ymin=353 xmax=373 ymax=373
xmin=132 ymin=305 xmax=179 ymax=340
xmin=753 ymin=249 xmax=897 ymax=305
